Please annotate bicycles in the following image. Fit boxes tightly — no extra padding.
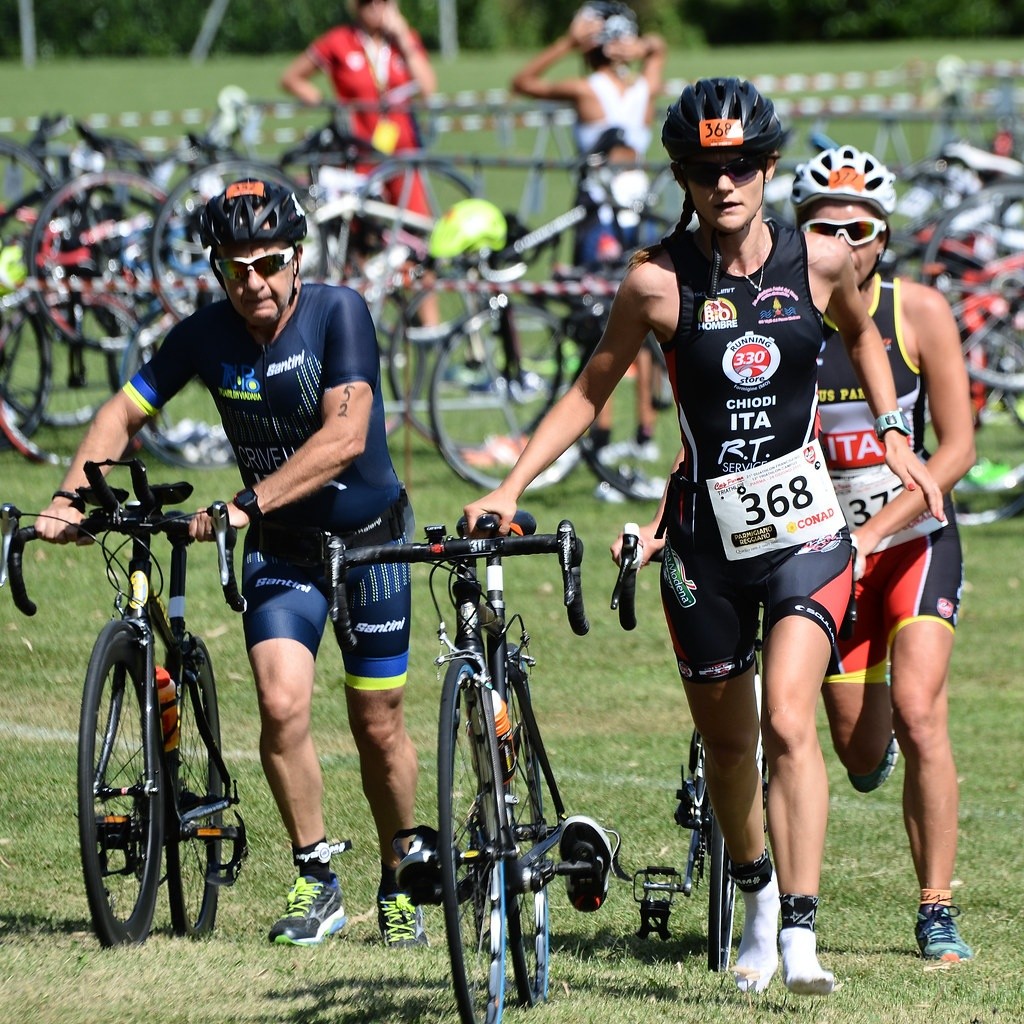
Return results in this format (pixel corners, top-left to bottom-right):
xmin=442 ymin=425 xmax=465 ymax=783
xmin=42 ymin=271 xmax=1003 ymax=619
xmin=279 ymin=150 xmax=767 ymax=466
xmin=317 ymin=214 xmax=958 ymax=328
xmin=1 ymin=103 xmax=1024 ymax=536
xmin=1 ymin=458 xmax=251 ymax=956
xmin=317 ymin=507 xmax=607 ymax=1024
xmin=608 ymin=521 xmax=775 ymax=975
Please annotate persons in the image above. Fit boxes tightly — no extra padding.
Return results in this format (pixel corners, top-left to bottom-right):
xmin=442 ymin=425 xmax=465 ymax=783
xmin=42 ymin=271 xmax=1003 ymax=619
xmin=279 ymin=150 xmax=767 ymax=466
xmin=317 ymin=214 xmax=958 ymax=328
xmin=458 ymin=75 xmax=946 ymax=994
xmin=510 ymin=1 xmax=669 ymax=453
xmin=35 ymin=177 xmax=430 ymax=960
xmin=278 ymin=1 xmax=436 ymax=323
xmin=788 ymin=142 xmax=977 ymax=962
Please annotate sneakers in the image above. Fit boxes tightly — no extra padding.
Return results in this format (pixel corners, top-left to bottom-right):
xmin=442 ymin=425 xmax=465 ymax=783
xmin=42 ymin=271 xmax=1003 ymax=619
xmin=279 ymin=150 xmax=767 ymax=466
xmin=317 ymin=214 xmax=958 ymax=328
xmin=376 ymin=891 xmax=429 ymax=948
xmin=849 ymin=730 xmax=899 ymax=792
xmin=267 ymin=871 xmax=346 ymax=946
xmin=914 ymin=897 xmax=971 ymax=960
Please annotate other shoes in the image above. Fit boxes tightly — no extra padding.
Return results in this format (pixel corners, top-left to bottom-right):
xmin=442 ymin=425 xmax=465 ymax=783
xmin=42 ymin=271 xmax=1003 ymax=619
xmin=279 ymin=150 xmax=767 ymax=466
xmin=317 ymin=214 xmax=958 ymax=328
xmin=625 ymin=443 xmax=660 ymax=459
xmin=558 ymin=815 xmax=613 ymax=912
xmin=393 ymin=826 xmax=446 ymax=894
xmin=598 ymin=444 xmax=616 ymax=466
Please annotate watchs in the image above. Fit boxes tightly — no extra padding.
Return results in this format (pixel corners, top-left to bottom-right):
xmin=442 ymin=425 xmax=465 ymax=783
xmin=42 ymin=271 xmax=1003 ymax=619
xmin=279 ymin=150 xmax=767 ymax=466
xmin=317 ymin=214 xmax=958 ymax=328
xmin=874 ymin=409 xmax=912 ymax=441
xmin=232 ymin=486 xmax=266 ymax=523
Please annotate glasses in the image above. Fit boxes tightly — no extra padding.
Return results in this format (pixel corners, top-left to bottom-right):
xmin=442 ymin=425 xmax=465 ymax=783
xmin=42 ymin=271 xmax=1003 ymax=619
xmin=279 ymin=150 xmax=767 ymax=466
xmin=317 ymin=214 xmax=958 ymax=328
xmin=215 ymin=244 xmax=296 ymax=281
xmin=800 ymin=218 xmax=888 ymax=246
xmin=675 ymin=150 xmax=769 ymax=187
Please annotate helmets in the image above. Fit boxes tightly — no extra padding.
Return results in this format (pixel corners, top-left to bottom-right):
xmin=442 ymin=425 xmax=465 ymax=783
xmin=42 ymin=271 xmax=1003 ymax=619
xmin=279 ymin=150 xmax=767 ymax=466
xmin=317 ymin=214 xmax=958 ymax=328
xmin=428 ymin=197 xmax=507 ymax=256
xmin=663 ymin=77 xmax=784 ymax=165
xmin=788 ymin=144 xmax=898 ymax=217
xmin=198 ymin=177 xmax=307 ymax=248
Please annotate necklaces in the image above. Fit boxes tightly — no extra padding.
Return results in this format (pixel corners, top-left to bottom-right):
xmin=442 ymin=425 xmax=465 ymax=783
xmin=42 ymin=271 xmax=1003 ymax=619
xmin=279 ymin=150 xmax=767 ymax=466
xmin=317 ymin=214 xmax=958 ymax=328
xmin=722 ymin=228 xmax=768 ymax=291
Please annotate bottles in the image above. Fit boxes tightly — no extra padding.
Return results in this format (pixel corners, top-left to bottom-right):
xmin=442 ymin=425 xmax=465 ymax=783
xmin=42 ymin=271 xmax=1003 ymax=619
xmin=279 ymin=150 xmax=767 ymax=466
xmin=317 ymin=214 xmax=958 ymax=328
xmin=155 ymin=664 xmax=180 ymax=751
xmin=466 ymin=694 xmax=517 ymax=783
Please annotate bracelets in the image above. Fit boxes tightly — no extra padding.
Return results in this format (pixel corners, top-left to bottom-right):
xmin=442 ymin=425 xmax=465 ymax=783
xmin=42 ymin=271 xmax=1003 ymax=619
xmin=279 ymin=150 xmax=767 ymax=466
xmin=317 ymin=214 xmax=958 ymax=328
xmin=51 ymin=490 xmax=78 ymax=502
